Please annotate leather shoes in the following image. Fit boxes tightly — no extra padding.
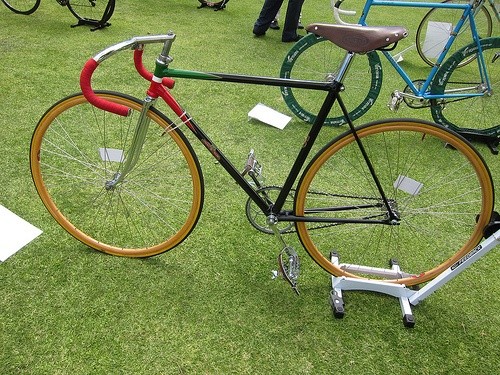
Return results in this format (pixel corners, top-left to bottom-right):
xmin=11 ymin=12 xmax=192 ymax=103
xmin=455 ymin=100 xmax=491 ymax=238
xmin=284 ymin=34 xmax=304 ymax=41
xmin=252 ymin=28 xmax=265 ymax=35
xmin=270 ymin=20 xmax=280 ymax=29
xmin=298 ymin=22 xmax=304 ymax=28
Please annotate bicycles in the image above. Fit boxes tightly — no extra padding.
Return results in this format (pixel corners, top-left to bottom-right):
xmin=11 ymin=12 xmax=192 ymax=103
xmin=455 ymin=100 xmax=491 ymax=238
xmin=29 ymin=21 xmax=495 ymax=294
xmin=2 ymin=0 xmax=116 ymax=25
xmin=282 ymin=0 xmax=500 ymax=142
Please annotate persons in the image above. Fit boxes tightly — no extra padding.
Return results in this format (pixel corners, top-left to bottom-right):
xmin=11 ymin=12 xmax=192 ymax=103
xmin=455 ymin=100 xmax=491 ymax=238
xmin=252 ymin=0 xmax=305 ymax=42
xmin=269 ymin=6 xmax=304 ymax=30
